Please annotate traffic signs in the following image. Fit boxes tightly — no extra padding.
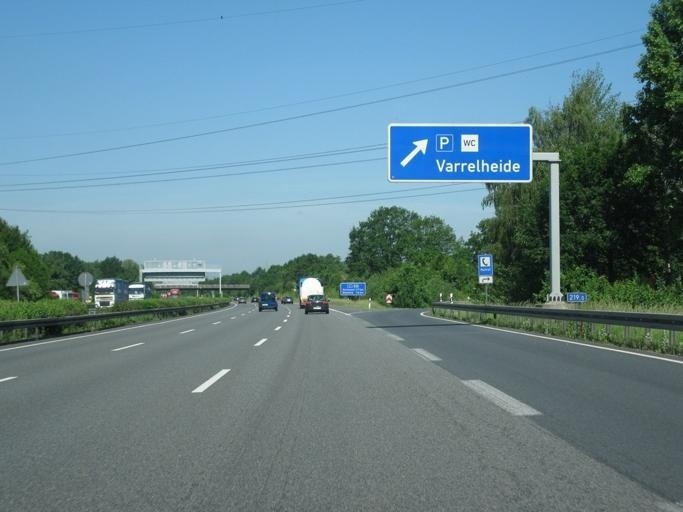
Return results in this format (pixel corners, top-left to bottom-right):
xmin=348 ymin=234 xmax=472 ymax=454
xmin=477 ymin=254 xmax=493 ymax=276
xmin=386 ymin=122 xmax=534 ymax=185
xmin=339 ymin=282 xmax=366 ymax=296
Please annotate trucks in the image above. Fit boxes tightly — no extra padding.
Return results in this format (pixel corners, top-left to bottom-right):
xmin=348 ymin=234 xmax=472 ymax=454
xmin=93 ymin=277 xmax=183 ymax=311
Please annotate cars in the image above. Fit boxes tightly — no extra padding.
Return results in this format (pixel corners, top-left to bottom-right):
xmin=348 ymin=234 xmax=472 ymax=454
xmin=232 ymin=277 xmax=330 ymax=315
